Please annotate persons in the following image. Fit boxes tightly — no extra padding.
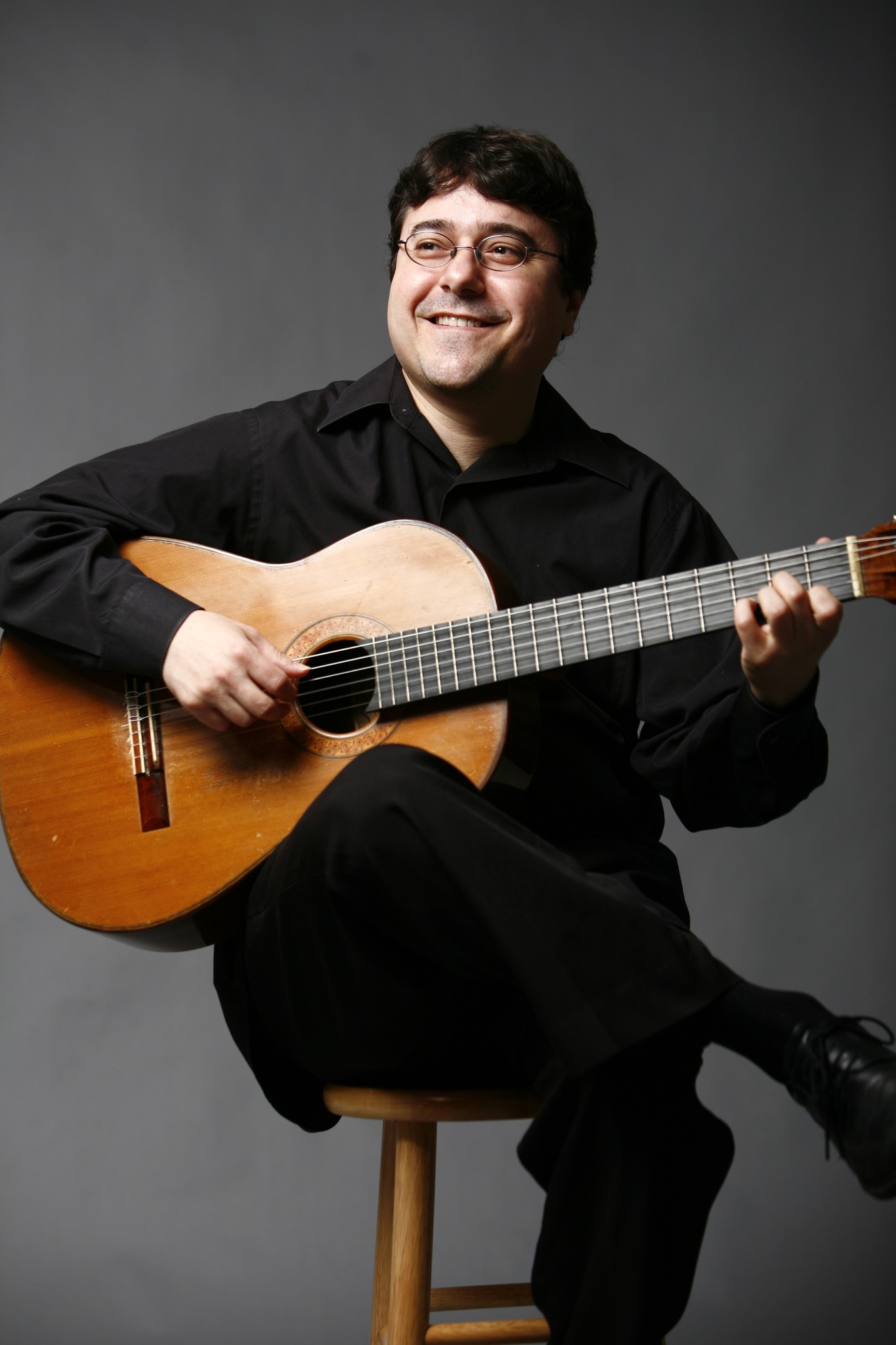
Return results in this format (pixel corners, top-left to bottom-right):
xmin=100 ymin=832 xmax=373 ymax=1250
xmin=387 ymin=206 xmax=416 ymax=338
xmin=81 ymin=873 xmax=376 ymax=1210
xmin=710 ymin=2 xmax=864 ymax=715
xmin=0 ymin=124 xmax=896 ymax=1345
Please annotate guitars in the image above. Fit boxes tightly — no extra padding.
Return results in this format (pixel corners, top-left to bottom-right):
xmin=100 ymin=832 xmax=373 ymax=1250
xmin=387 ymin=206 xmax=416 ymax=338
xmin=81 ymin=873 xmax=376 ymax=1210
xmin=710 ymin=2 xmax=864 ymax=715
xmin=1 ymin=517 xmax=896 ymax=954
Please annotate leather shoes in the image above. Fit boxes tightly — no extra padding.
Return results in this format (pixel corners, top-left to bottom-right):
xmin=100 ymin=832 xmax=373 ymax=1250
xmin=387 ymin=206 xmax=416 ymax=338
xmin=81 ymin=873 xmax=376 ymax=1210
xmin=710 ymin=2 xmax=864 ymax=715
xmin=768 ymin=992 xmax=895 ymax=1200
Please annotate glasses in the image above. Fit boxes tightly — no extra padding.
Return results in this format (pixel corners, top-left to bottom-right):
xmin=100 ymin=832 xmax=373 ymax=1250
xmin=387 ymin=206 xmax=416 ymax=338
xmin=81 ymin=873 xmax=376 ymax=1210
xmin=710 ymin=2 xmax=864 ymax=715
xmin=394 ymin=231 xmax=563 ymax=272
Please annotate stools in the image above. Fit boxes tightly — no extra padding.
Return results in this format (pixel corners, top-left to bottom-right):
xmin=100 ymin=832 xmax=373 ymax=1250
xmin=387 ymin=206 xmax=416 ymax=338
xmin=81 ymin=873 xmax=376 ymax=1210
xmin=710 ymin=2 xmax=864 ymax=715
xmin=322 ymin=1084 xmax=556 ymax=1344
